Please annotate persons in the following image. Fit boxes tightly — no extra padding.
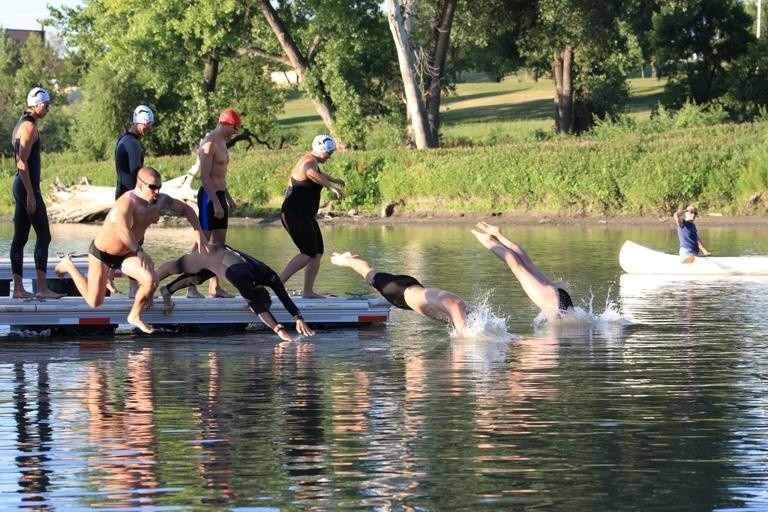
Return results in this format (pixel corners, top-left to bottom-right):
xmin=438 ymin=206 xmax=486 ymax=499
xmin=142 ymin=243 xmax=316 ymax=342
xmin=107 ymin=104 xmax=157 ymax=299
xmin=471 ymin=223 xmax=573 ymax=320
xmin=11 ymin=86 xmax=66 ymax=299
xmin=331 ymin=251 xmax=479 ymax=341
xmin=276 ymin=134 xmax=344 ymax=299
xmin=55 ymin=166 xmax=211 ymax=335
xmin=186 ymin=108 xmax=242 ymax=298
xmin=674 ymin=205 xmax=712 ymax=257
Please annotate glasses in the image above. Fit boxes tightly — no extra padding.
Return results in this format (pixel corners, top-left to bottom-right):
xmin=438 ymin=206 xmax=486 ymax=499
xmin=688 ymin=210 xmax=698 ymax=215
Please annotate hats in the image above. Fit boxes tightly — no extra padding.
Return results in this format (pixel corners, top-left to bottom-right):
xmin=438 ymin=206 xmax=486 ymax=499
xmin=311 ymin=132 xmax=335 ymax=154
xmin=131 ymin=105 xmax=154 ymax=126
xmin=23 ymin=82 xmax=51 ymax=109
xmin=220 ymin=108 xmax=241 ymax=127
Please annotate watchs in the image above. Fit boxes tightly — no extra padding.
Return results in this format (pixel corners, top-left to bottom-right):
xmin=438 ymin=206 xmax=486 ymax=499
xmin=273 ymin=324 xmax=284 ymax=332
xmin=133 ymin=246 xmax=143 ymax=254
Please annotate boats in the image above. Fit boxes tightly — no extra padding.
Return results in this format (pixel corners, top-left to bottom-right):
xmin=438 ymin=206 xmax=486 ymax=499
xmin=617 ymin=240 xmax=767 ymax=280
